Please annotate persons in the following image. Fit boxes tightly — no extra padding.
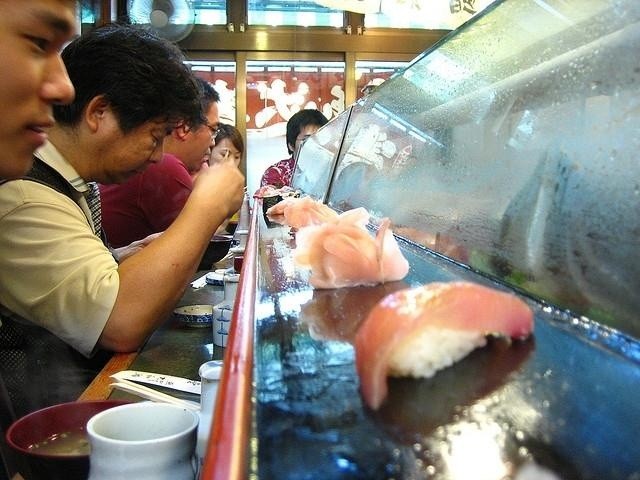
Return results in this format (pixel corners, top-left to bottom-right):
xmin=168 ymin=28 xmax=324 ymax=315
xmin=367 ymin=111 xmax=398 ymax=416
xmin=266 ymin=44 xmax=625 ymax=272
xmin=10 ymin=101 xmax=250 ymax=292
xmin=97 ymin=75 xmax=222 ymax=250
xmin=260 ymin=108 xmax=330 ymax=189
xmin=0 ymin=1 xmax=82 ymax=184
xmin=207 ymin=121 xmax=244 ymax=169
xmin=0 ymin=22 xmax=245 ymax=479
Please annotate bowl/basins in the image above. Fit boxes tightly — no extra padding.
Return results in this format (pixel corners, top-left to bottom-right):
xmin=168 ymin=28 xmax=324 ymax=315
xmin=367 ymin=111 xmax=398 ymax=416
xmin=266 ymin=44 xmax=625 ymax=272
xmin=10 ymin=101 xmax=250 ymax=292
xmin=3 ymin=400 xmax=133 ymax=479
xmin=196 ymin=235 xmax=232 ymax=271
xmin=172 ymin=303 xmax=218 ymax=328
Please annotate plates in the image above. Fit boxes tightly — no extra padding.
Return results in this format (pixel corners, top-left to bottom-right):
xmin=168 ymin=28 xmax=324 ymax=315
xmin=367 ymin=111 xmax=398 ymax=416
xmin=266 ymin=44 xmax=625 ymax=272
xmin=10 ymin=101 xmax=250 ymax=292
xmin=205 ymin=269 xmax=233 ymax=286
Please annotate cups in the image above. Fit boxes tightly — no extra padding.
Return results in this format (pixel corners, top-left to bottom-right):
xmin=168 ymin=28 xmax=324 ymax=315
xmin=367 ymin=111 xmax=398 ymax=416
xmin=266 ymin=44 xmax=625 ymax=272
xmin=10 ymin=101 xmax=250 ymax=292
xmin=85 ymin=401 xmax=199 ymax=479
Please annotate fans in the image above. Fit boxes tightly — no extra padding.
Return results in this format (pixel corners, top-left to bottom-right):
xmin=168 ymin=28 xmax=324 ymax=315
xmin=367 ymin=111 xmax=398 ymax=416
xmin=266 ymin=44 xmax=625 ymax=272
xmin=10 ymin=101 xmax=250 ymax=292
xmin=126 ymin=0 xmax=196 ymax=43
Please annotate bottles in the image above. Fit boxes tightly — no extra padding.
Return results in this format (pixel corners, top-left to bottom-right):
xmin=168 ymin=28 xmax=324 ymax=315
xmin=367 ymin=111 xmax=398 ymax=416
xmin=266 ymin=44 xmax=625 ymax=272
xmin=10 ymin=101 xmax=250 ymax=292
xmin=211 ymin=272 xmax=242 ymax=347
xmin=194 ymin=359 xmax=235 ymax=461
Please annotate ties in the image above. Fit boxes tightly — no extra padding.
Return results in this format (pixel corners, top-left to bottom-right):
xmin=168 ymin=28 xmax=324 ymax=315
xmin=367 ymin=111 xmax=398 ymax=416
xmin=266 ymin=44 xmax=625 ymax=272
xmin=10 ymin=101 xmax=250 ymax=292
xmin=86 ymin=180 xmax=104 ymax=242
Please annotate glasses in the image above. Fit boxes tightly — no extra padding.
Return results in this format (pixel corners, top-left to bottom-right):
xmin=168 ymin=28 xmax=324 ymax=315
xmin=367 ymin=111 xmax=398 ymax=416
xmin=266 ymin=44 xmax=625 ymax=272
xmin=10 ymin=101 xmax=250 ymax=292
xmin=190 ymin=116 xmax=221 ymax=140
